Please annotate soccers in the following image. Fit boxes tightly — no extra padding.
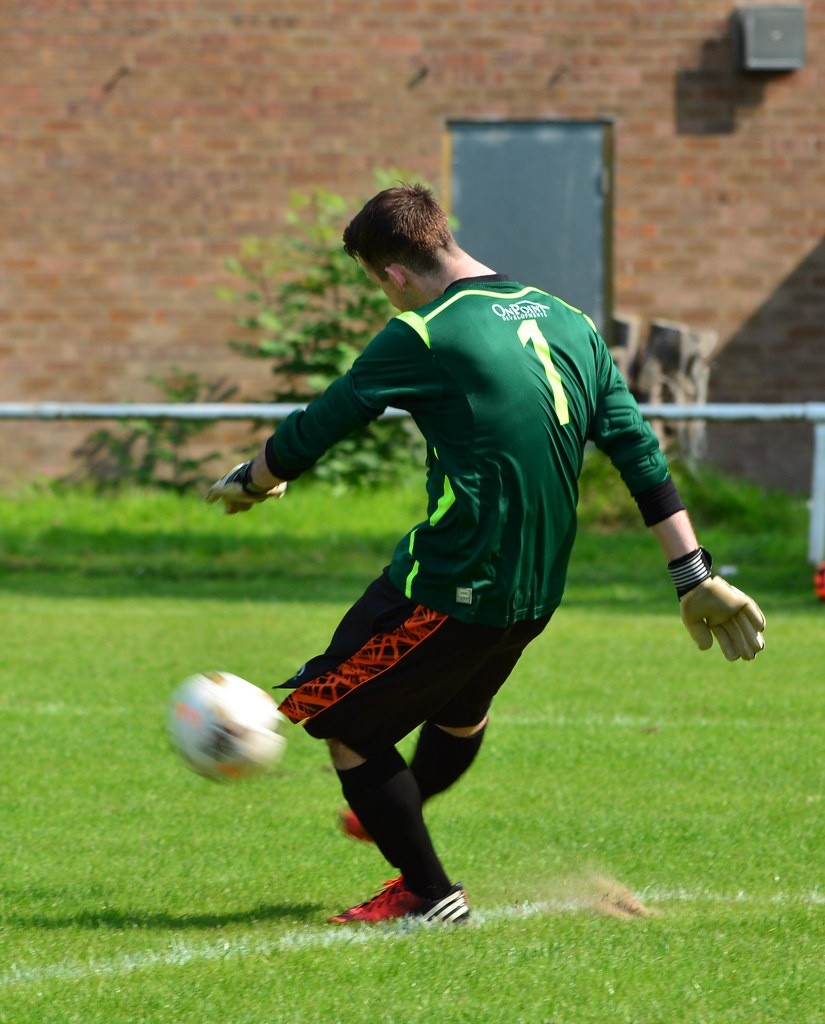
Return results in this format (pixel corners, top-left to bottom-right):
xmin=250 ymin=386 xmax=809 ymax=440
xmin=163 ymin=670 xmax=288 ymax=786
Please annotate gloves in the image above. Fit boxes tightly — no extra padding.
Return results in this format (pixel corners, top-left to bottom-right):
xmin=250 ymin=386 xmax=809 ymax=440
xmin=205 ymin=457 xmax=288 ymax=514
xmin=668 ymin=545 xmax=766 ymax=662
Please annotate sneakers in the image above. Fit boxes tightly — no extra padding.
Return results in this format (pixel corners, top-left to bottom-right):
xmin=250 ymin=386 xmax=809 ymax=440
xmin=338 ymin=808 xmax=374 ymax=843
xmin=326 ymin=874 xmax=469 ymax=924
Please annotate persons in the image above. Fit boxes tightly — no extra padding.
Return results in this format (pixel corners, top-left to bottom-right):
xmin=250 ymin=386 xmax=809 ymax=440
xmin=207 ymin=182 xmax=766 ymax=926
